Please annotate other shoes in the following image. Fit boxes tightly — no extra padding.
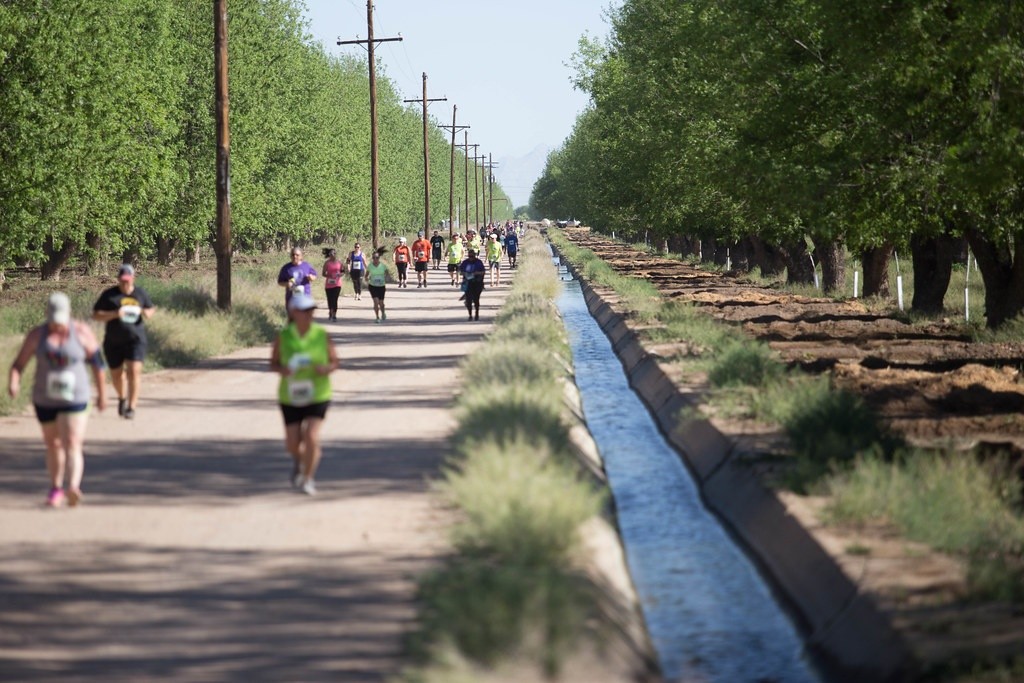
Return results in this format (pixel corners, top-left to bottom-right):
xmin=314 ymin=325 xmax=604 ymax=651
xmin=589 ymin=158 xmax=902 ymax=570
xmin=456 ymin=282 xmax=460 ymax=287
xmin=67 ymin=487 xmax=80 ymax=506
xmin=433 ymin=266 xmax=440 ymax=270
xmin=451 ymin=279 xmax=455 ymax=286
xmin=403 ymin=282 xmax=407 ymax=288
xmin=424 ymin=280 xmax=427 ymax=288
xmin=355 ymin=294 xmax=361 ymax=301
xmin=301 ymin=482 xmax=317 ymax=496
xmin=329 ymin=313 xmax=337 ymax=321
xmin=398 ymin=283 xmax=403 ymax=288
xmin=118 ymin=398 xmax=133 ymax=420
xmin=46 ymin=490 xmax=63 ymax=507
xmin=512 ymin=262 xmax=515 ymax=268
xmin=474 ymin=315 xmax=479 ymax=321
xmin=375 ymin=319 xmax=380 ymax=323
xmin=509 ymin=267 xmax=513 ymax=269
xmin=381 ymin=312 xmax=386 ymax=320
xmin=417 ymin=283 xmax=421 ymax=288
xmin=291 ymin=462 xmax=303 ymax=488
xmin=468 ymin=317 xmax=473 ymax=321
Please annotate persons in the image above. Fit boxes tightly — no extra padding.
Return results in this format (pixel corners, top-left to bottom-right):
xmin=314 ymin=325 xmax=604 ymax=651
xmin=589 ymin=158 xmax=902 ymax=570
xmin=444 ymin=234 xmax=464 ymax=287
xmin=484 ymin=234 xmax=503 ymax=287
xmin=366 ymin=246 xmax=389 ymax=323
xmin=392 ymin=237 xmax=413 ymax=288
xmin=90 ymin=263 xmax=158 ymax=422
xmin=411 ymin=231 xmax=433 ymax=287
xmin=460 ymin=250 xmax=485 ymax=320
xmin=321 ymin=248 xmax=345 ymax=321
xmin=465 ymin=229 xmax=482 ymax=258
xmin=8 ymin=290 xmax=106 ymax=508
xmin=430 ymin=231 xmax=446 ymax=270
xmin=480 ymin=219 xmax=524 ymax=245
xmin=272 ymin=292 xmax=340 ymax=496
xmin=278 ymin=247 xmax=318 ymax=322
xmin=346 ymin=243 xmax=368 ymax=300
xmin=504 ymin=227 xmax=520 ymax=269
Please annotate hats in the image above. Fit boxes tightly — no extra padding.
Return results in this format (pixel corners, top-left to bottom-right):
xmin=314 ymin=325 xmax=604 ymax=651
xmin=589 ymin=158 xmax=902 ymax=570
xmin=289 ymin=293 xmax=318 ymax=311
xmin=119 ymin=265 xmax=134 ymax=283
xmin=47 ymin=292 xmax=70 ymax=327
xmin=399 ymin=237 xmax=404 ymax=242
xmin=489 ymin=234 xmax=497 ymax=239
